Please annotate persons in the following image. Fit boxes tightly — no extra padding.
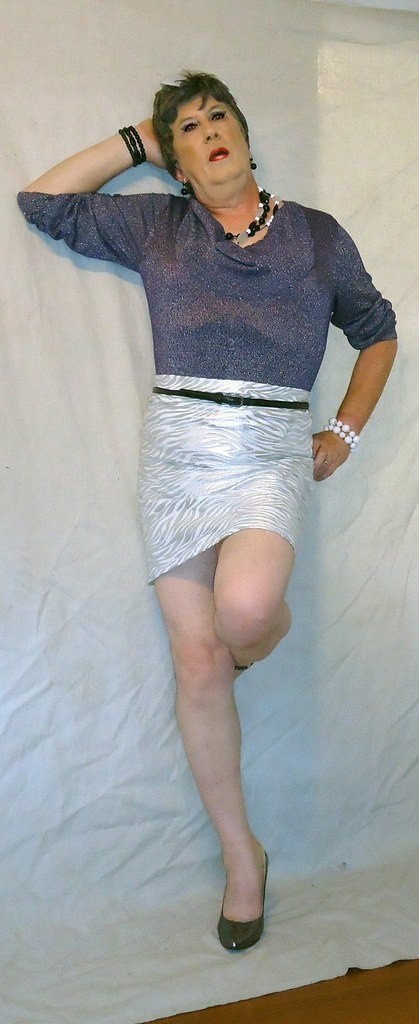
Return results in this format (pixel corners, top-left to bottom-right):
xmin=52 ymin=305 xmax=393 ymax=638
xmin=17 ymin=72 xmax=398 ymax=951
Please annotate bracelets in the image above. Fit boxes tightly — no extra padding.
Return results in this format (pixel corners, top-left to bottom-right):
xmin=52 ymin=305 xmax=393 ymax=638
xmin=118 ymin=126 xmax=146 ymax=167
xmin=324 ymin=417 xmax=360 ymax=453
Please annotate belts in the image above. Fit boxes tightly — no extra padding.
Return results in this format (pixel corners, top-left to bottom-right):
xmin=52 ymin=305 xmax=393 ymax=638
xmin=153 ymin=387 xmax=309 ymax=409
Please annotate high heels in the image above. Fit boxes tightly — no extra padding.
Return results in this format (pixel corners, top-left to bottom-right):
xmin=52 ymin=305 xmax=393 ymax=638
xmin=217 ymin=850 xmax=269 ymax=951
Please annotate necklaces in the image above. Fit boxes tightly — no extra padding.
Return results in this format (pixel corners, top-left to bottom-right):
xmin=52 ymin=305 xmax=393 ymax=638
xmin=224 ymin=186 xmax=279 ymax=244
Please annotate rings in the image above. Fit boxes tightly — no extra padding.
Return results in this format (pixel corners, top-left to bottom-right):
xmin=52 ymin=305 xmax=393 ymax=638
xmin=312 ymin=455 xmax=316 ymax=460
xmin=324 ymin=460 xmax=330 ymax=465
